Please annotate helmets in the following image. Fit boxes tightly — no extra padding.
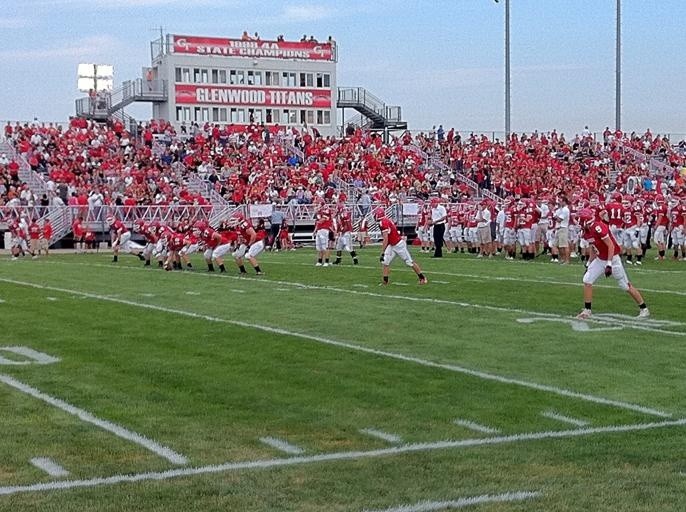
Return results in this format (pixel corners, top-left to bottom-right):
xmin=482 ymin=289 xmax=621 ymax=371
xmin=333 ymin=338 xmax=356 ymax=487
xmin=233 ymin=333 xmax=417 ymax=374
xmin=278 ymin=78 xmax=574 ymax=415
xmin=579 ymin=195 xmax=666 ymax=210
xmin=374 ymin=208 xmax=386 ymax=221
xmin=7 ymin=219 xmax=14 ymax=227
xmin=518 ymin=198 xmax=530 ymax=209
xmin=504 ymin=196 xmax=515 ymax=204
xmin=432 ymin=198 xmax=439 ymax=203
xmin=193 ymin=221 xmax=207 ymax=234
xmin=134 ymin=218 xmax=171 ymax=243
xmin=578 ymin=209 xmax=592 ymax=222
xmin=480 ymin=201 xmax=488 ymax=205
xmin=230 ymin=212 xmax=245 ymax=226
xmin=106 ymin=214 xmax=115 ymax=225
xmin=336 ymin=204 xmax=344 ymax=212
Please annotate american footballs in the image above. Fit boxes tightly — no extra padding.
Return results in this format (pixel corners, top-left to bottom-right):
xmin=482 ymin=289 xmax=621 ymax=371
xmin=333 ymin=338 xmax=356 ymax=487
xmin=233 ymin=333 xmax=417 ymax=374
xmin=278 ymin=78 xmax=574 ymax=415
xmin=165 ymin=263 xmax=173 ymax=270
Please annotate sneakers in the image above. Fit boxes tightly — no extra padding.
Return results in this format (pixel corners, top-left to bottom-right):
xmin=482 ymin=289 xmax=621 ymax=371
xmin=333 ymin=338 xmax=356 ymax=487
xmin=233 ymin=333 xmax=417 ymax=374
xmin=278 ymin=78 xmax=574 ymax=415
xmin=477 ymin=250 xmax=535 ymax=261
xmin=546 ymin=252 xmax=578 ymax=265
xmin=624 ymin=259 xmax=642 ymax=267
xmin=315 ymin=261 xmax=340 ymax=267
xmin=11 ymin=251 xmax=49 ymax=260
xmin=420 ymin=277 xmax=427 ymax=284
xmin=379 ymin=281 xmax=390 ymax=286
xmin=239 ymin=271 xmax=248 ymax=275
xmin=656 ymin=256 xmax=686 ymax=262
xmin=576 ymin=308 xmax=592 ymax=320
xmin=420 ymin=245 xmax=478 ymax=257
xmin=256 ymin=271 xmax=263 ymax=275
xmin=207 ymin=268 xmax=215 ymax=272
xmin=143 ymin=260 xmax=192 ymax=271
xmin=640 ymin=307 xmax=651 ymax=318
xmin=220 ymin=270 xmax=226 ymax=274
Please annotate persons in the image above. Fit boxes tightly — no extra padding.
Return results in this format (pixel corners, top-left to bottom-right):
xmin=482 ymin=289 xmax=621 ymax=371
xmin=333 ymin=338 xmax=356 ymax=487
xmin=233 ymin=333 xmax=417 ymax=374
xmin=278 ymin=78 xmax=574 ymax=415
xmin=2 ymin=110 xmax=685 ymax=284
xmin=573 ymin=207 xmax=652 ymax=321
xmin=278 ymin=35 xmax=284 ymax=41
xmin=301 ymin=36 xmax=307 ymax=43
xmin=309 ymin=36 xmax=318 ymax=42
xmin=242 ymin=31 xmax=253 ymax=41
xmin=326 ymin=36 xmax=334 ymax=45
xmin=146 ymin=69 xmax=154 ymax=93
xmin=251 ymin=32 xmax=260 ymax=42
xmin=87 ymin=89 xmax=111 ymax=109
